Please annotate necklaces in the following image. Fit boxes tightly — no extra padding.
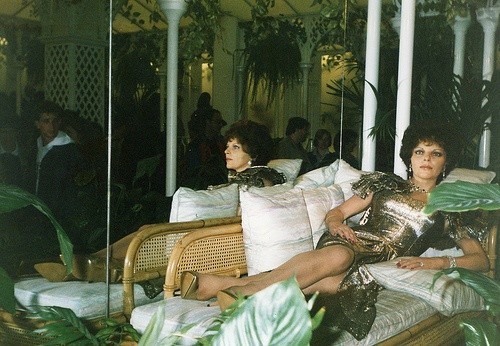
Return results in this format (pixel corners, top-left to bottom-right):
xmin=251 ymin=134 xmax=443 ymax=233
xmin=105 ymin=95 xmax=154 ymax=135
xmin=409 ymin=179 xmax=431 ymax=196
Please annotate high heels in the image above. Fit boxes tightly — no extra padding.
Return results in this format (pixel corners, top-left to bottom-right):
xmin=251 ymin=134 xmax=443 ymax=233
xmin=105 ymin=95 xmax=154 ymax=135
xmin=207 ymin=286 xmax=240 ymax=313
xmin=173 ymin=270 xmax=200 ymax=299
xmin=33 ymin=262 xmax=76 ymax=281
xmin=60 ymin=252 xmax=124 ymax=285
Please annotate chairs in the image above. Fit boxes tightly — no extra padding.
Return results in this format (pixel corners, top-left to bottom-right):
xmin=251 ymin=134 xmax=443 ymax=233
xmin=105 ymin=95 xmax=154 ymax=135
xmin=120 ymin=158 xmax=500 ymax=346
xmin=0 ymin=161 xmax=339 ymax=346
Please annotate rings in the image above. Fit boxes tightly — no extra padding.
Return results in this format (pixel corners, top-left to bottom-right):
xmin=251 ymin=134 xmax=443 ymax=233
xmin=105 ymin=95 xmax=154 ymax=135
xmin=418 ymin=261 xmax=423 ymax=267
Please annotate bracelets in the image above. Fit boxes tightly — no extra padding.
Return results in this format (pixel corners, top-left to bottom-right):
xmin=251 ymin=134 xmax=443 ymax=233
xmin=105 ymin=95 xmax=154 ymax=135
xmin=443 ymin=255 xmax=456 ymax=269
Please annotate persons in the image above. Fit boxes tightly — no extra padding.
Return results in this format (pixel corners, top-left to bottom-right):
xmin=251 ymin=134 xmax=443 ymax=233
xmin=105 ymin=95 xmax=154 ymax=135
xmin=186 ymin=93 xmax=226 ymax=143
xmin=174 ymin=118 xmax=491 ymax=341
xmin=272 ymin=116 xmax=361 ymax=179
xmin=26 ymin=101 xmax=78 ymax=219
xmin=33 ymin=118 xmax=287 ymax=300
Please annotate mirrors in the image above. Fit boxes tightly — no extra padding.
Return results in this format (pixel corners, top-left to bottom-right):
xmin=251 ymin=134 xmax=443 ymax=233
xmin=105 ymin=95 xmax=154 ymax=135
xmin=0 ymin=0 xmax=500 ymax=284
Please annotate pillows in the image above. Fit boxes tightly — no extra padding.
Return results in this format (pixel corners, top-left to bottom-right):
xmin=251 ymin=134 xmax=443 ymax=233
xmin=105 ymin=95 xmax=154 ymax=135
xmin=362 ymin=253 xmax=490 ymax=318
xmin=163 ymin=158 xmax=304 ymax=261
xmin=238 ymin=159 xmax=381 ymax=275
